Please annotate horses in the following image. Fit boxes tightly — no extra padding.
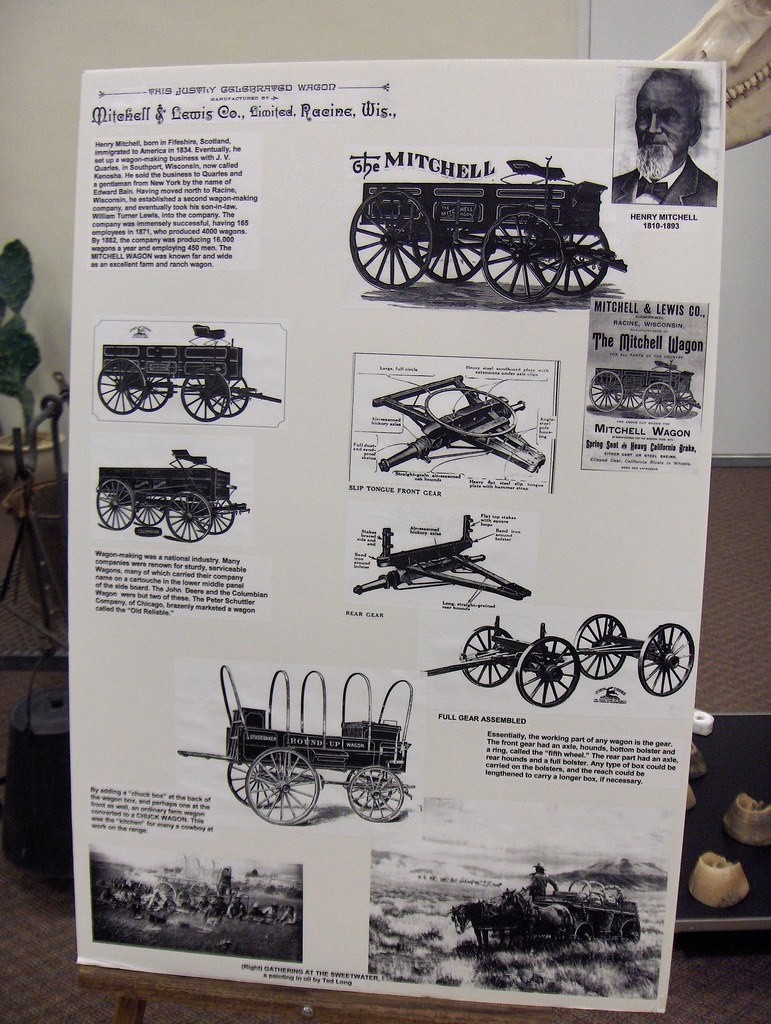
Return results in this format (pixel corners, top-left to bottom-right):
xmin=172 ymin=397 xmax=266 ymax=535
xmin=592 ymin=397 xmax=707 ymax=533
xmin=497 ymin=887 xmax=574 ymax=952
xmin=447 ymin=899 xmax=519 ymax=948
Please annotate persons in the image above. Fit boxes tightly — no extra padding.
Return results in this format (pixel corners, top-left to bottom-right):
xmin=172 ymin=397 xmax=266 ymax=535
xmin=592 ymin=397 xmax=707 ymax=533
xmin=612 ymin=70 xmax=719 ymax=208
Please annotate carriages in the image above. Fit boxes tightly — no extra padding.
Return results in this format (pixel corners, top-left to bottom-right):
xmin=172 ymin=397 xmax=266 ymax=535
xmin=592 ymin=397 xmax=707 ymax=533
xmin=450 ymin=880 xmax=641 ymax=948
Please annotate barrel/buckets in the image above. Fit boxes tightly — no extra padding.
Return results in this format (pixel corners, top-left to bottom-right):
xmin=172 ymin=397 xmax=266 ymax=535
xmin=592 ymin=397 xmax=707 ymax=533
xmin=3 ymin=643 xmax=73 ymax=878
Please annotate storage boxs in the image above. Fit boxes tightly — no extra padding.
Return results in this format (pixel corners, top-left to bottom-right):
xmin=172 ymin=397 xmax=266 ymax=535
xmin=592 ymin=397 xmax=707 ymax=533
xmin=2 ymin=478 xmax=68 ymax=616
xmin=1 ymin=684 xmax=73 ymax=881
